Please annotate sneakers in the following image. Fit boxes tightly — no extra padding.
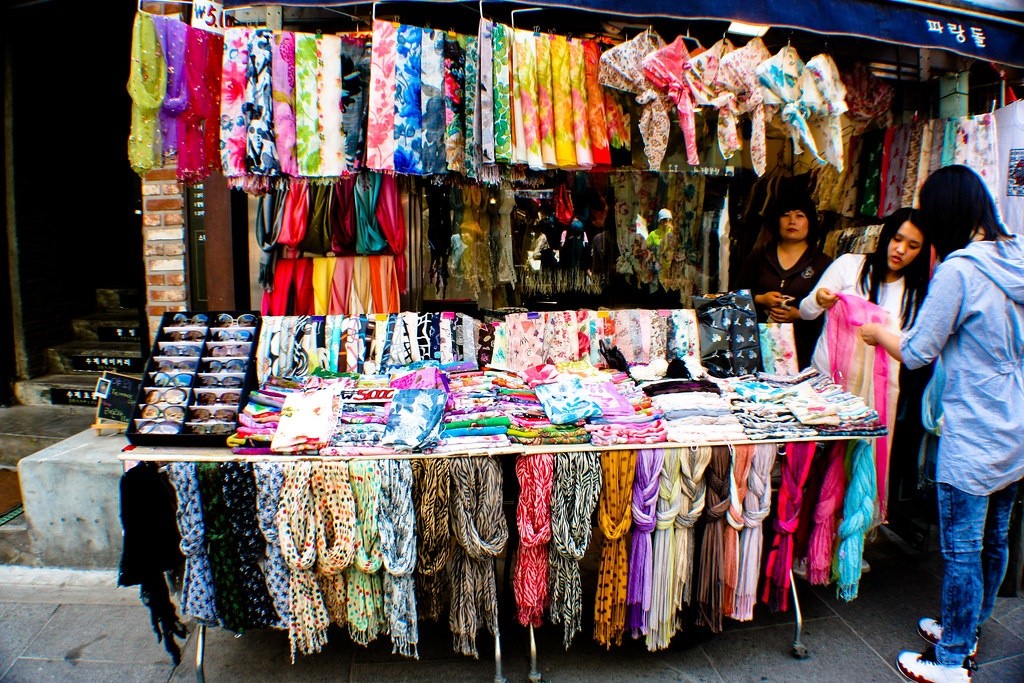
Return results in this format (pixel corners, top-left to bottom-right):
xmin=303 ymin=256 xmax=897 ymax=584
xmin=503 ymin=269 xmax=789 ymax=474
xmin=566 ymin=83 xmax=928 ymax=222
xmin=917 ymin=618 xmax=980 ymax=658
xmin=896 ymin=649 xmax=978 ymax=683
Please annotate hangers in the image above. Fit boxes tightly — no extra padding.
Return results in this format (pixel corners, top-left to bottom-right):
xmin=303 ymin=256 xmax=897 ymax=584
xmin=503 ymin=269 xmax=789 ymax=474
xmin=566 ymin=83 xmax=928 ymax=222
xmin=135 ymin=0 xmax=551 ymax=38
xmin=645 ymin=25 xmax=830 ymax=62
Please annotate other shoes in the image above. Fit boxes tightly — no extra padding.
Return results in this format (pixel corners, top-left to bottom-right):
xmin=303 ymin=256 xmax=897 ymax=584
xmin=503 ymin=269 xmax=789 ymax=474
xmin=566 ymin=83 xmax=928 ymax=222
xmin=862 ymin=559 xmax=870 ymax=572
xmin=792 ymin=556 xmax=808 ymax=577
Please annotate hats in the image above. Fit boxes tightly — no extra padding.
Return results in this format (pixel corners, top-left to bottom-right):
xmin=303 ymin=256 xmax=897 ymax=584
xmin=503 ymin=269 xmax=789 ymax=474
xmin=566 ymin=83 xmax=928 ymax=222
xmin=658 ymin=208 xmax=673 ymax=222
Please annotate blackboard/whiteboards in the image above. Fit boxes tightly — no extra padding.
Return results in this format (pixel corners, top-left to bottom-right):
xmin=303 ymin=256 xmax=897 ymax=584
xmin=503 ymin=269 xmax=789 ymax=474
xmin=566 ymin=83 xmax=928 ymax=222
xmin=98 ymin=372 xmax=142 ymax=423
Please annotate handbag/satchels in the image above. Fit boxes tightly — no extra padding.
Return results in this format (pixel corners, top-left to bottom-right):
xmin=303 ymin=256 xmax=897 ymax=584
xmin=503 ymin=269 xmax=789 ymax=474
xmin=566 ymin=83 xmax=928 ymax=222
xmin=689 ymin=288 xmax=766 ymax=378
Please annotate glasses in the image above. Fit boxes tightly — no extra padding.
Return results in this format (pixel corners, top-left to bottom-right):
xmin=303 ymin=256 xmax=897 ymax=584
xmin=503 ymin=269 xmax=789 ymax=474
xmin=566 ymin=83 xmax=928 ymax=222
xmin=136 ymin=314 xmax=256 ymax=435
xmin=661 ymin=219 xmax=672 ymax=222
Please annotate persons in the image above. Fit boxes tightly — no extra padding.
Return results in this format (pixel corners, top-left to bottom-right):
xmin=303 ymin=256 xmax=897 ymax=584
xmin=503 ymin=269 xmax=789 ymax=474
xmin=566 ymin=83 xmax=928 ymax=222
xmin=530 ymin=183 xmax=606 ymax=274
xmin=646 ymin=208 xmax=675 ymax=259
xmin=798 ymin=203 xmax=931 ymax=573
xmin=859 ymin=164 xmax=1024 ymax=683
xmin=744 ymin=196 xmax=835 ymax=373
